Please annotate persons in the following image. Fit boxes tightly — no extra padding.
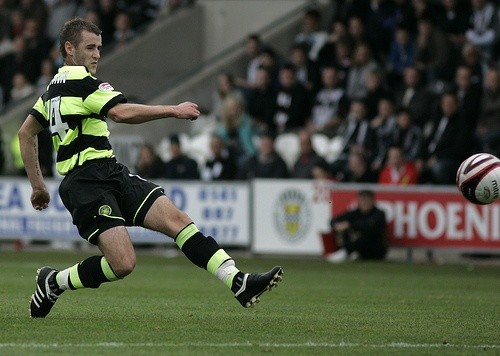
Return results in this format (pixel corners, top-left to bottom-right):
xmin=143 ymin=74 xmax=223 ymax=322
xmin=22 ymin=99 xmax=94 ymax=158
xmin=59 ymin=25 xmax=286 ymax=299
xmin=0 ymin=0 xmax=195 ymax=110
xmin=18 ymin=17 xmax=285 ymax=318
xmin=326 ymin=189 xmax=388 ymax=263
xmin=133 ymin=0 xmax=499 ymax=180
xmin=11 ymin=133 xmax=27 ymax=177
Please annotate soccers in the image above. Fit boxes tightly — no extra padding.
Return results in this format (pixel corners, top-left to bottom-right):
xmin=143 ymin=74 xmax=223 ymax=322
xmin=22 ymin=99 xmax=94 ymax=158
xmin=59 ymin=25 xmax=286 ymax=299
xmin=455 ymin=151 xmax=500 ymax=205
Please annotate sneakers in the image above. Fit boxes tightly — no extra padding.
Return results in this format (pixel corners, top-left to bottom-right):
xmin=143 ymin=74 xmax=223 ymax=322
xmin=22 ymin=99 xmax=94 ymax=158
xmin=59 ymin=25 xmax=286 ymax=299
xmin=29 ymin=266 xmax=59 ymax=317
xmin=235 ymin=265 xmax=285 ymax=309
